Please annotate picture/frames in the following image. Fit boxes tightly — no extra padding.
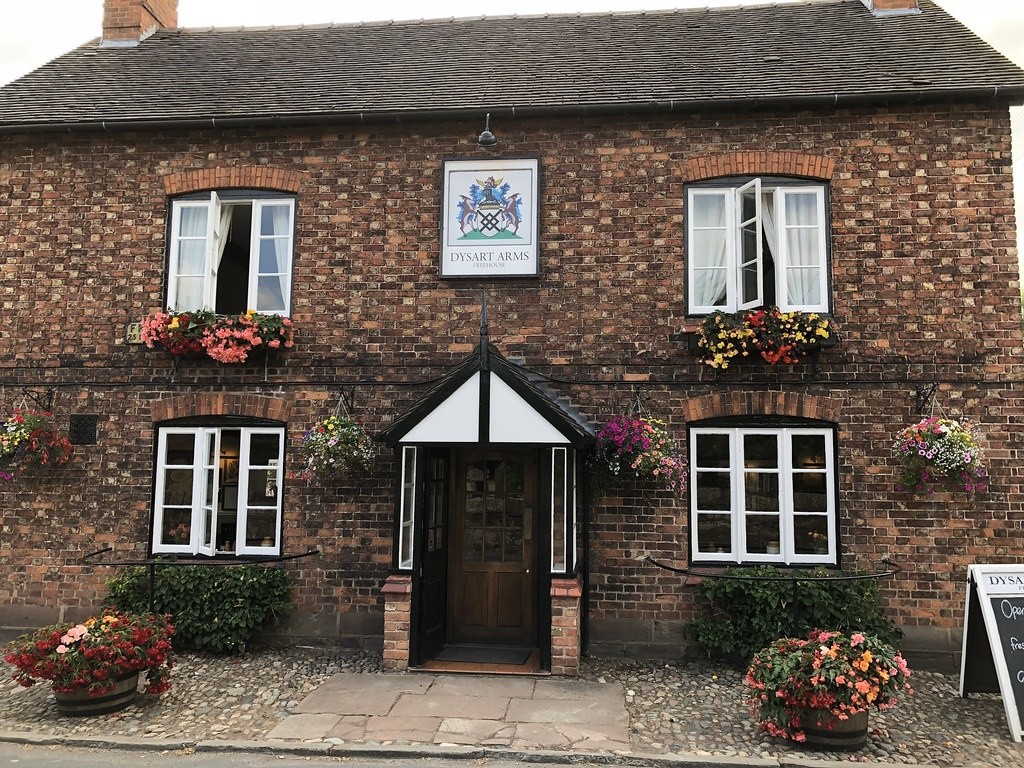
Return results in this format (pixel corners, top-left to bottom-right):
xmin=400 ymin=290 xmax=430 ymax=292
xmin=221 ymin=485 xmax=237 ymax=511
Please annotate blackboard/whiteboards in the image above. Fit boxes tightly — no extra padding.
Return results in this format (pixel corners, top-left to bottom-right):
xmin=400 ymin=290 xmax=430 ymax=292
xmin=960 ymin=563 xmax=1024 ymax=732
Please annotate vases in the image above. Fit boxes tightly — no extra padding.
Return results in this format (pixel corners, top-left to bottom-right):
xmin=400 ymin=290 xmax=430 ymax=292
xmin=779 ymin=707 xmax=870 ymax=751
xmin=174 ymin=538 xmax=188 ymax=544
xmin=54 ymin=672 xmax=144 ymax=717
xmin=813 ymin=545 xmax=828 ymax=553
xmin=328 ymin=466 xmax=364 ymax=495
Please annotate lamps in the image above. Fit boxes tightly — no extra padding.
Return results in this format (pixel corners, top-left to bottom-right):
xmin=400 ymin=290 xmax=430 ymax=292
xmin=475 ymin=114 xmax=497 ymax=148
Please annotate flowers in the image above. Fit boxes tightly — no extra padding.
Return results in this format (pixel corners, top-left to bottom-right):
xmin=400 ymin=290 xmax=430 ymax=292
xmin=0 ymin=407 xmax=70 ymax=482
xmin=693 ymin=306 xmax=830 ymax=370
xmin=1 ymin=607 xmax=175 ymax=698
xmin=596 ymin=402 xmax=685 ymax=492
xmin=888 ymin=413 xmax=991 ymax=494
xmin=140 ymin=306 xmax=297 ymax=363
xmin=744 ymin=628 xmax=916 ymax=742
xmin=168 ymin=523 xmax=190 ymax=538
xmin=807 ymin=530 xmax=827 ymax=547
xmin=296 ymin=415 xmax=377 ymax=477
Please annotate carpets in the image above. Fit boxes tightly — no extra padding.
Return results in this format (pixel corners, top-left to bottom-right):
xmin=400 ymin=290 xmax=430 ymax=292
xmin=431 ymin=646 xmax=533 ymax=664
